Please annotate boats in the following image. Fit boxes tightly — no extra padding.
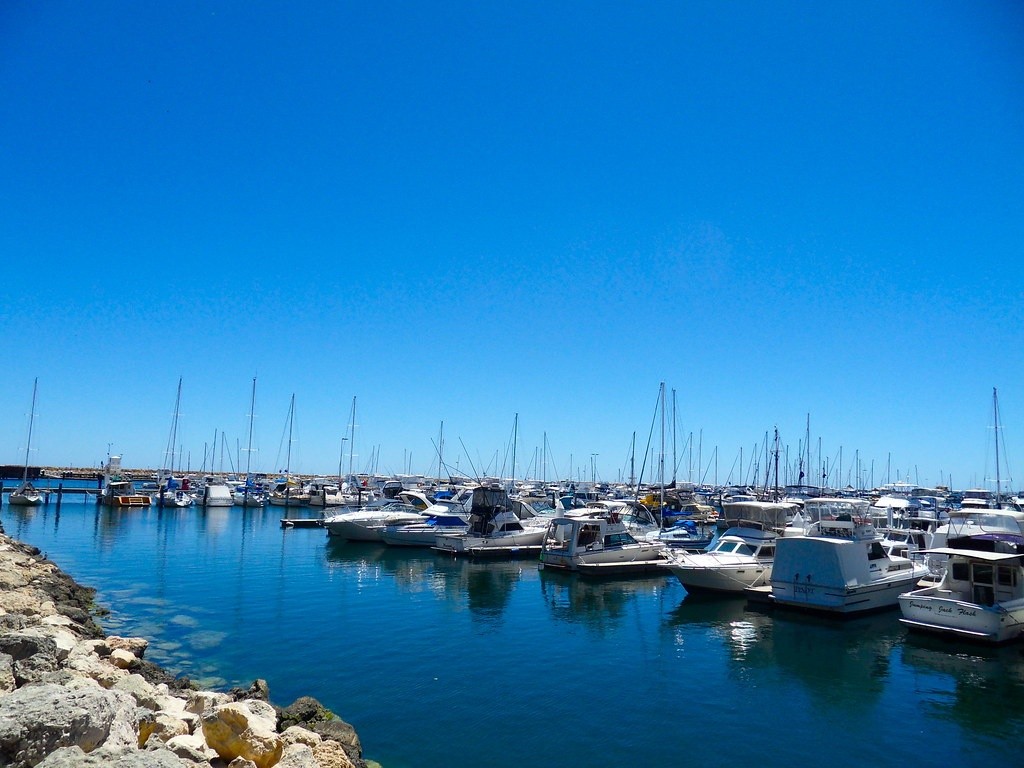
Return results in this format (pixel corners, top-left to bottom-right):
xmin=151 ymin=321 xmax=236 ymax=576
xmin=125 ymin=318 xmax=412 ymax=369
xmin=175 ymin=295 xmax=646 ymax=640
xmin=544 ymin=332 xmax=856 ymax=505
xmin=769 ymin=521 xmax=929 ymax=615
xmin=896 ymin=533 xmax=1024 ymax=641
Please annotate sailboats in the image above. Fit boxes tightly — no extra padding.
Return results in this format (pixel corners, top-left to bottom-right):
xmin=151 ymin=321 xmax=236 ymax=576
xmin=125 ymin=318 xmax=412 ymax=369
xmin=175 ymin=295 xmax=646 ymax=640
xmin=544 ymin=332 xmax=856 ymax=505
xmin=101 ymin=378 xmax=1023 ymax=592
xmin=8 ymin=377 xmax=46 ymax=505
